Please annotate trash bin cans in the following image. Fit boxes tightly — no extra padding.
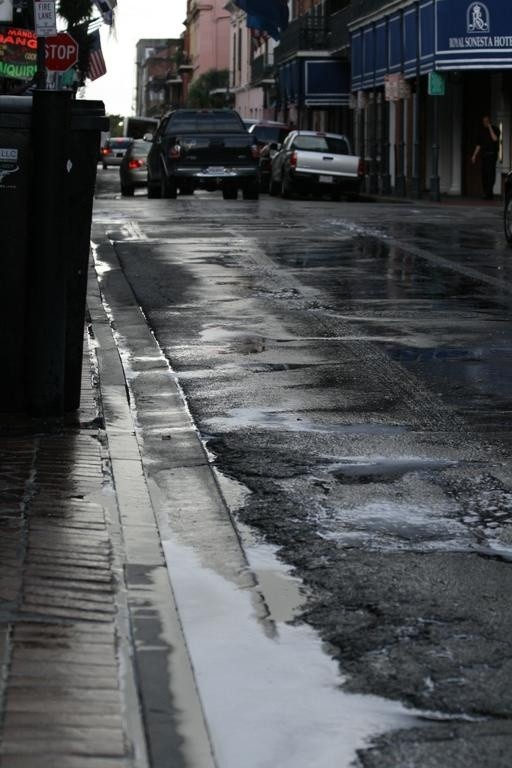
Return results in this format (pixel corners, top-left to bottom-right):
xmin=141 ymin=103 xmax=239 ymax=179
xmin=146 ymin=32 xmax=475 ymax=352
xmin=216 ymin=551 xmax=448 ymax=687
xmin=0 ymin=89 xmax=111 ymax=415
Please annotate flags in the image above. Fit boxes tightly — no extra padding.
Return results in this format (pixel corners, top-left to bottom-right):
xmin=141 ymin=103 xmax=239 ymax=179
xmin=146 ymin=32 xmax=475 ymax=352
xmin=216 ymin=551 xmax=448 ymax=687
xmin=87 ymin=29 xmax=107 ymax=81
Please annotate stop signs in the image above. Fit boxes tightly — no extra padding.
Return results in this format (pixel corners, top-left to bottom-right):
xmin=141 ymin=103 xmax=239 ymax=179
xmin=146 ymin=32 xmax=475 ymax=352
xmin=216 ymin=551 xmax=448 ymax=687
xmin=44 ymin=33 xmax=80 ymax=72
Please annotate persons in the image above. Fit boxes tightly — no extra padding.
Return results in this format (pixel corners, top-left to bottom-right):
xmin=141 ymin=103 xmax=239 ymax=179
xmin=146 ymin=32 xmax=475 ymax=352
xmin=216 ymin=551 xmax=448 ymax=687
xmin=471 ymin=114 xmax=501 ymax=200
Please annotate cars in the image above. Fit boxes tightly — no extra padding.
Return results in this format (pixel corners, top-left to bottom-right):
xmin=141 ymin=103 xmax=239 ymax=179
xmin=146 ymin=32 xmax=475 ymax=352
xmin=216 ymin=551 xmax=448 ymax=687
xmin=102 ymin=104 xmax=368 ymax=202
